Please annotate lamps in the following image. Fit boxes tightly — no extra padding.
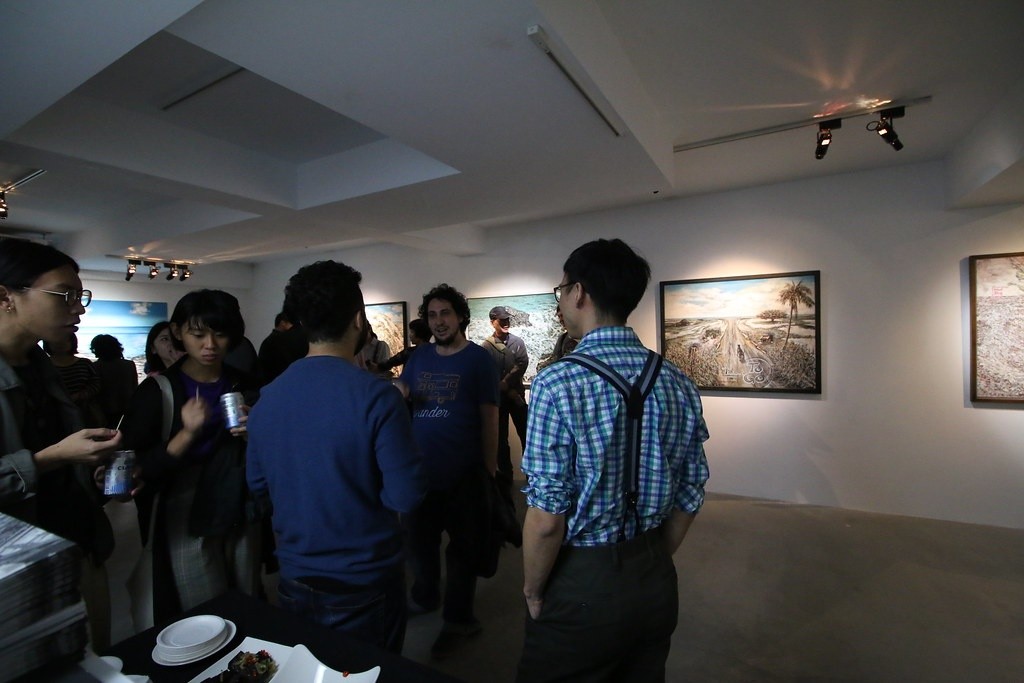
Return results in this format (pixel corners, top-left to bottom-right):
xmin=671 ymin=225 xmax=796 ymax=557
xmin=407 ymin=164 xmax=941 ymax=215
xmin=177 ymin=264 xmax=194 ymax=281
xmin=164 ymin=263 xmax=179 ymax=281
xmin=866 ymin=105 xmax=905 ymax=151
xmin=0 ymin=187 xmax=8 ymax=219
xmin=144 ymin=260 xmax=159 ymax=280
xmin=126 ymin=260 xmax=141 ymax=281
xmin=815 ymin=118 xmax=842 ymax=160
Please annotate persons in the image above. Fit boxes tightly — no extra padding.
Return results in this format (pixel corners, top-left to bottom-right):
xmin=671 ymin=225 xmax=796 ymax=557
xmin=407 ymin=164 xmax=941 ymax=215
xmin=361 ymin=320 xmax=390 ymax=364
xmin=513 ymin=238 xmax=710 ymax=683
xmin=481 ymin=306 xmax=529 ymax=484
xmin=144 ymin=321 xmax=187 ymax=377
xmin=538 ymin=304 xmax=579 ymax=372
xmin=43 ymin=331 xmax=101 ymax=411
xmin=0 ymin=240 xmax=145 ymax=652
xmin=382 ymin=318 xmax=432 ymax=370
xmin=224 ymin=312 xmax=309 ymax=386
xmin=245 ymin=259 xmax=423 ymax=654
xmin=122 ymin=289 xmax=267 ymax=612
xmin=395 ymin=283 xmax=500 ymax=653
xmin=91 ymin=335 xmax=138 ymax=419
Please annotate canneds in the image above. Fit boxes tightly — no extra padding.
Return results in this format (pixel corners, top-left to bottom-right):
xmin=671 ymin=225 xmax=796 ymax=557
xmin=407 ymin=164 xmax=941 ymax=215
xmin=219 ymin=391 xmax=248 ymax=429
xmin=103 ymin=450 xmax=136 ymax=496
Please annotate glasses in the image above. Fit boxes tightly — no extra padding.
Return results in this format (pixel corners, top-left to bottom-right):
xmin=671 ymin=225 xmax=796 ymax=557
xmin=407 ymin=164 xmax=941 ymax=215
xmin=554 ymin=282 xmax=588 ymax=302
xmin=21 ymin=287 xmax=92 ymax=306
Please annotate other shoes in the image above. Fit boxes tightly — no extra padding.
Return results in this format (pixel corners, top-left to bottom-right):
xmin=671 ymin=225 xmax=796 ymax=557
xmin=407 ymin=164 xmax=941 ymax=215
xmin=430 ymin=627 xmax=468 ymax=657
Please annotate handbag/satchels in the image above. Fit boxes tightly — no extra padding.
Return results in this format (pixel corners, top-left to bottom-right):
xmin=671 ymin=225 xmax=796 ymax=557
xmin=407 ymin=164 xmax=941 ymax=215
xmin=126 ymin=548 xmax=156 ymax=634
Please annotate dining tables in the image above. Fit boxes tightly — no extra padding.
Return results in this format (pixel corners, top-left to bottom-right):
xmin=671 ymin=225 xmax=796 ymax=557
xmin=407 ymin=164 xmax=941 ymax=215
xmin=95 ymin=589 xmax=466 ymax=683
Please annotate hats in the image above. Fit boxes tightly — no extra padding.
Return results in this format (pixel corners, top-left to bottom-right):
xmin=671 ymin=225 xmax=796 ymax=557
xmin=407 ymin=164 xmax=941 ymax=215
xmin=489 ymin=306 xmax=512 ymax=319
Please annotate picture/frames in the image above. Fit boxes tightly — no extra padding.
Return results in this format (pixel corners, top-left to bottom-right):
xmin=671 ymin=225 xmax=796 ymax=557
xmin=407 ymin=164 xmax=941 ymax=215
xmin=969 ymin=252 xmax=1024 ymax=403
xmin=364 ymin=301 xmax=407 ymax=378
xmin=467 ymin=293 xmax=567 ymax=386
xmin=660 ymin=269 xmax=821 ymax=394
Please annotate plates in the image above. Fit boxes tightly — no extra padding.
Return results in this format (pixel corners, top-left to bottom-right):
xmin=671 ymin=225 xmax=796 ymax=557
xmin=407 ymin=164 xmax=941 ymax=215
xmin=268 ymin=644 xmax=380 ymax=683
xmin=188 ymin=636 xmax=294 ymax=683
xmin=152 ymin=614 xmax=237 ymax=666
